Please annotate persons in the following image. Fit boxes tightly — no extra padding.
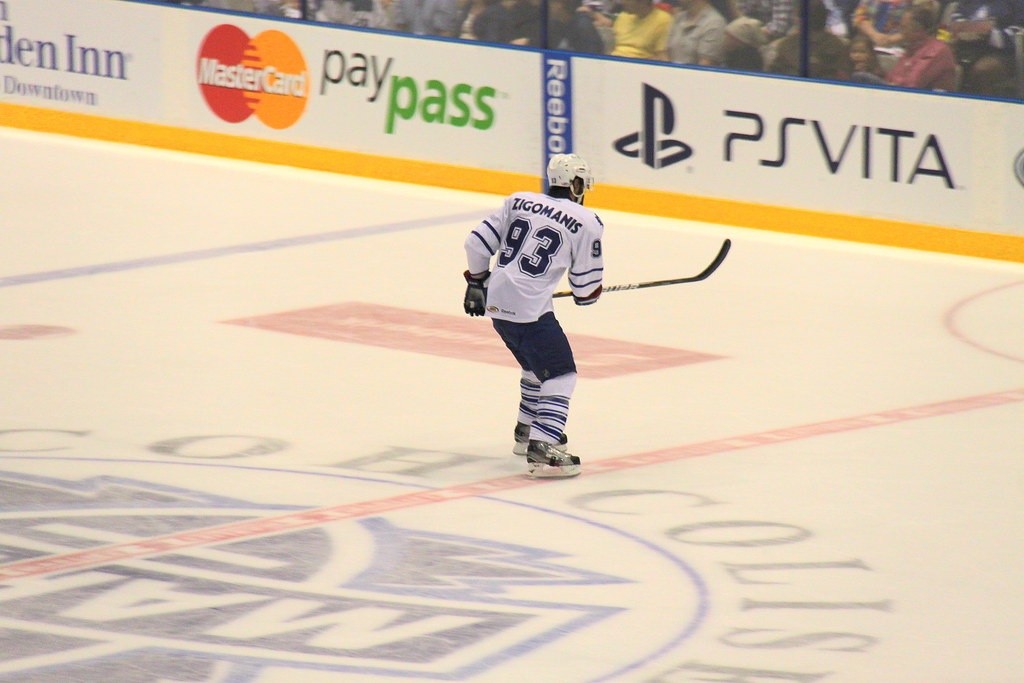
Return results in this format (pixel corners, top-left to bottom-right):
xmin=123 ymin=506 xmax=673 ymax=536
xmin=174 ymin=1 xmax=1024 ymax=101
xmin=462 ymin=151 xmax=606 ymax=467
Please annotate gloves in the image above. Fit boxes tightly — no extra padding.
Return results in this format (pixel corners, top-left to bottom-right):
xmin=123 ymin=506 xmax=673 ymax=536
xmin=574 ymin=285 xmax=603 ymax=305
xmin=463 ymin=268 xmax=492 ymax=316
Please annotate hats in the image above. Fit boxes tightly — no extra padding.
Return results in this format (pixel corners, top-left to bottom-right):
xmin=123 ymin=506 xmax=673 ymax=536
xmin=725 ymin=16 xmax=762 ymax=49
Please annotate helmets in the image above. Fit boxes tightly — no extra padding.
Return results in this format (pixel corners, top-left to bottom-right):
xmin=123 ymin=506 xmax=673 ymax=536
xmin=546 ymin=153 xmax=591 ymax=187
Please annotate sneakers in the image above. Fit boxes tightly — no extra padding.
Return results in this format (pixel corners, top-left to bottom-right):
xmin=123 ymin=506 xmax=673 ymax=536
xmin=527 ymin=439 xmax=581 ymax=477
xmin=512 ymin=425 xmax=568 ymax=455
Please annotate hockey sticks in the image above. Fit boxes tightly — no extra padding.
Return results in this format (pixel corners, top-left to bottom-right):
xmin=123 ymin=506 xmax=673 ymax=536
xmin=552 ymin=238 xmax=731 ymax=298
